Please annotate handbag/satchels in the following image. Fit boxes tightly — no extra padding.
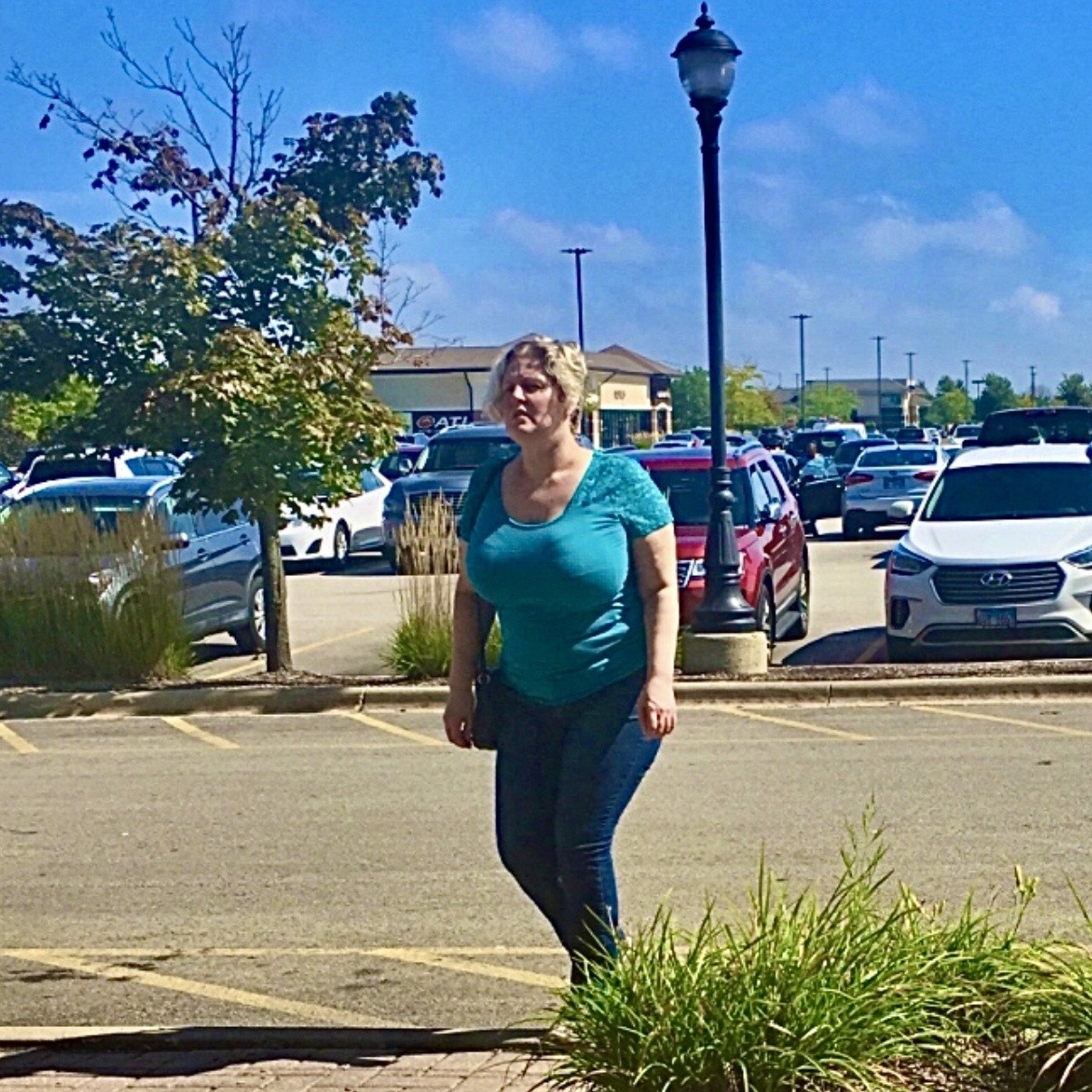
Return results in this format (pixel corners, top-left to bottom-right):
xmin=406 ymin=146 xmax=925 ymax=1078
xmin=472 ymin=667 xmax=507 ymax=752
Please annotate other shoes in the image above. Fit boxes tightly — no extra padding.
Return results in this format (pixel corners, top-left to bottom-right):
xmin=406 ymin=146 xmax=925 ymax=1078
xmin=541 ymin=936 xmax=633 ymax=1051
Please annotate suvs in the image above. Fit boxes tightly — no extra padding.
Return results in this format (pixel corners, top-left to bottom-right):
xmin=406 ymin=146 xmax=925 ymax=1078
xmin=601 ymin=442 xmax=811 ymax=668
xmin=382 ymin=423 xmax=594 ymax=576
xmin=881 ymin=444 xmax=1092 ymax=665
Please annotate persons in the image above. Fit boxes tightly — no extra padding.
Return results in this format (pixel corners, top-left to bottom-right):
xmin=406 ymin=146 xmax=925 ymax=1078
xmin=443 ymin=331 xmax=681 ymax=1050
xmin=798 ymin=444 xmax=828 ymax=536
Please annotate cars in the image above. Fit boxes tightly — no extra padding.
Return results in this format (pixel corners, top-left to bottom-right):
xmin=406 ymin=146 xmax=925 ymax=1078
xmin=650 ymin=405 xmax=1092 ymax=544
xmin=0 ymin=475 xmax=267 ymax=671
xmin=0 ymin=430 xmax=429 ymax=575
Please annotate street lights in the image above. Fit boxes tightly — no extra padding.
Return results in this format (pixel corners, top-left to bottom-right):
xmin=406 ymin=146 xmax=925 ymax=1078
xmin=961 ymin=359 xmax=972 ymax=425
xmin=789 ymin=313 xmax=814 ymax=430
xmin=560 ymin=247 xmax=594 ymax=353
xmin=867 ymin=335 xmax=888 ymax=434
xmin=665 ymin=0 xmax=761 ymax=637
xmin=972 ymin=380 xmax=987 ymax=420
xmin=904 ymin=351 xmax=918 ymax=428
xmin=823 ymin=365 xmax=833 ymax=421
